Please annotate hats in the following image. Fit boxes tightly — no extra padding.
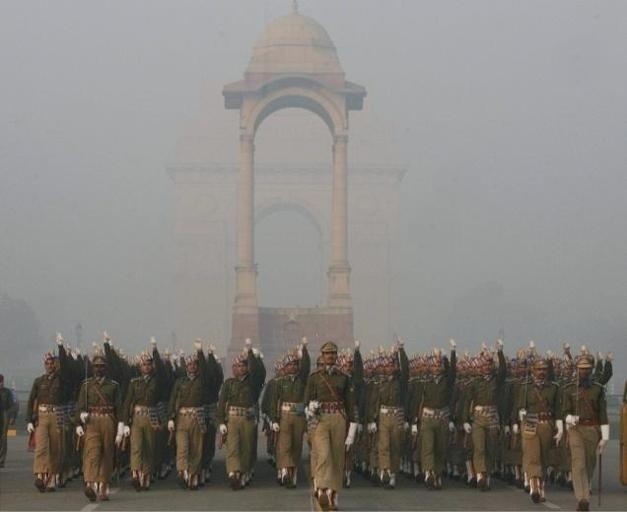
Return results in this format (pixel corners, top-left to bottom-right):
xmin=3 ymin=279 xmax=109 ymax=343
xmin=409 ymin=356 xmax=441 ymax=366
xmin=363 ymin=356 xmax=394 ymax=366
xmin=187 ymin=352 xmax=197 ymax=363
xmin=140 ymin=352 xmax=152 ymax=365
xmin=459 ymin=355 xmax=493 ymax=368
xmin=321 ymin=341 xmax=338 ymax=352
xmin=92 ymin=356 xmax=105 ymax=363
xmin=533 ymin=358 xmax=548 ymax=368
xmin=43 ymin=353 xmax=54 ymax=362
xmin=577 ymin=359 xmax=593 ymax=368
xmin=236 ymin=352 xmax=247 ymax=362
xmin=284 ymin=354 xmax=297 ymax=366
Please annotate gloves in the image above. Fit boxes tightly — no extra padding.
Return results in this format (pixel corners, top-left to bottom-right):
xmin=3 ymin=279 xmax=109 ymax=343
xmin=273 ymin=423 xmax=279 ymax=432
xmin=464 ymin=423 xmax=472 ymax=434
xmin=553 ymin=420 xmax=563 ymax=441
xmin=345 ymin=422 xmax=357 ymax=445
xmin=77 ymin=426 xmax=84 ymax=436
xmin=513 ymin=424 xmax=520 ymax=434
xmin=370 ymin=422 xmax=378 ymax=433
xmin=116 ymin=422 xmax=124 ymax=444
xmin=168 ymin=420 xmax=175 ymax=430
xmin=599 ymin=425 xmax=610 ymax=449
xmin=27 ymin=423 xmax=34 ymax=433
xmin=81 ymin=412 xmax=88 ymax=422
xmin=566 ymin=415 xmax=578 ymax=425
xmin=124 ymin=426 xmax=130 ymax=436
xmin=218 ymin=424 xmax=226 ymax=434
xmin=195 ymin=338 xmax=204 ymax=349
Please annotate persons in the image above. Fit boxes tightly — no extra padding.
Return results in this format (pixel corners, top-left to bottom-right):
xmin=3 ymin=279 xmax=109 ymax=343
xmin=75 ymin=332 xmax=131 ymax=502
xmin=218 ymin=339 xmax=258 ymax=490
xmin=620 ymin=379 xmax=627 ymax=486
xmin=167 ymin=338 xmax=217 ymax=490
xmin=25 ymin=334 xmax=84 ymax=494
xmin=0 ymin=374 xmax=17 ymax=467
xmin=305 ymin=340 xmax=364 ymax=511
xmin=447 ymin=339 xmax=506 ymax=492
xmin=545 ymin=344 xmax=614 ymax=512
xmin=123 ymin=336 xmax=176 ymax=492
xmin=407 ymin=339 xmax=457 ymax=490
xmin=261 ymin=339 xmax=311 ymax=490
xmin=492 ymin=342 xmax=564 ymax=503
xmin=354 ymin=337 xmax=410 ymax=491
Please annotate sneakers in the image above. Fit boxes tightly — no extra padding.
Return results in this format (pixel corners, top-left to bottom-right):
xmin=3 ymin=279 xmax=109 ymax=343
xmin=228 ymin=471 xmax=254 ymax=491
xmin=577 ymin=500 xmax=590 ymax=512
xmin=278 ymin=474 xmax=297 ymax=488
xmin=355 ymin=467 xmax=396 ymax=489
xmin=133 ymin=464 xmax=173 ymax=492
xmin=84 ymin=468 xmax=128 ymax=501
xmin=400 ymin=468 xmax=443 ymax=489
xmin=176 ymin=467 xmax=212 ymax=491
xmin=505 ymin=472 xmax=545 ymax=503
xmin=320 ymin=494 xmax=329 ymax=512
xmin=446 ymin=473 xmax=492 ymax=491
xmin=35 ymin=470 xmax=81 ymax=491
